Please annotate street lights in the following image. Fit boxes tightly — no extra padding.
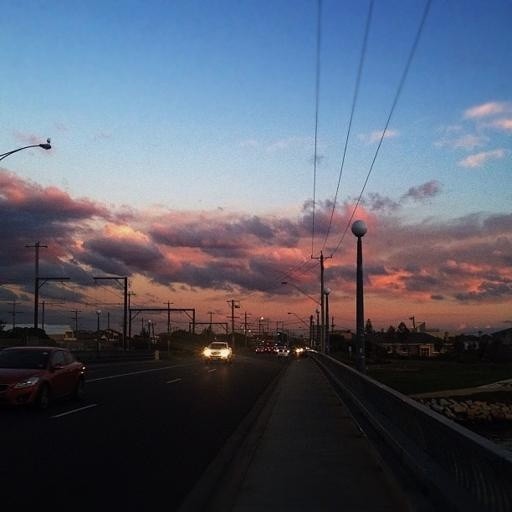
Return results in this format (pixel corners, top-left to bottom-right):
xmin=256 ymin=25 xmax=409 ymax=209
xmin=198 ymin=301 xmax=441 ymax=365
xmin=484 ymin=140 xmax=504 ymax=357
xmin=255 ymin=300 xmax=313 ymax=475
xmin=280 ymin=281 xmax=325 ymax=355
xmin=309 ymin=307 xmax=321 ymax=349
xmin=351 ymin=217 xmax=367 ymax=371
xmin=96 ymin=308 xmax=101 ymax=352
xmin=324 ymin=287 xmax=330 ymax=355
xmin=0 ymin=142 xmax=50 ymax=159
xmin=149 ymin=319 xmax=151 ymax=340
xmin=287 ymin=312 xmax=313 ymax=347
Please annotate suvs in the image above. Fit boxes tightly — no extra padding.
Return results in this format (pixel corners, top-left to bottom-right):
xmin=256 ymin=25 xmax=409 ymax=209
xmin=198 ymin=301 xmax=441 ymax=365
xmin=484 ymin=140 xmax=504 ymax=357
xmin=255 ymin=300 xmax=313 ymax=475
xmin=203 ymin=340 xmax=232 ymax=366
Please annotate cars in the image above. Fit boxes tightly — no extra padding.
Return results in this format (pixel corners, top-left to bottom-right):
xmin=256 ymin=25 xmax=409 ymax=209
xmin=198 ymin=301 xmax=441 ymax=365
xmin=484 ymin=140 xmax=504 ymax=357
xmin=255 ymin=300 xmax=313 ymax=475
xmin=255 ymin=344 xmax=308 ymax=357
xmin=0 ymin=346 xmax=88 ymax=411
xmin=150 ymin=335 xmax=159 ymax=344
